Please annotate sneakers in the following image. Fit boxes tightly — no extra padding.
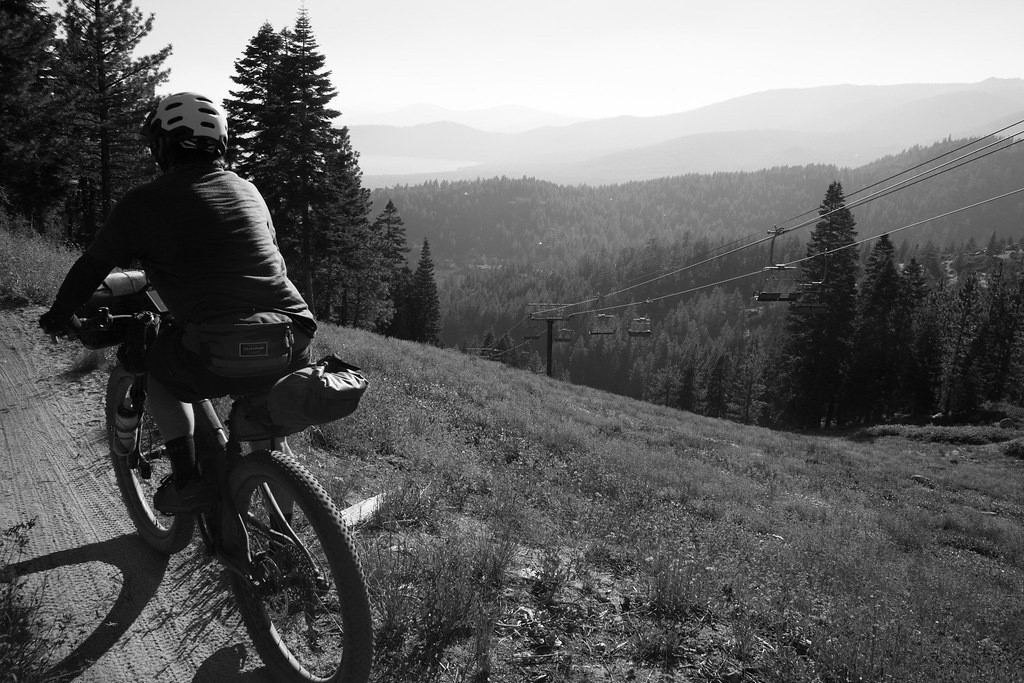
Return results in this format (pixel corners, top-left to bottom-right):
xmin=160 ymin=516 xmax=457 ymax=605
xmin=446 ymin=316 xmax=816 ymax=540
xmin=251 ymin=548 xmax=299 ymax=571
xmin=153 ymin=473 xmax=211 ymax=513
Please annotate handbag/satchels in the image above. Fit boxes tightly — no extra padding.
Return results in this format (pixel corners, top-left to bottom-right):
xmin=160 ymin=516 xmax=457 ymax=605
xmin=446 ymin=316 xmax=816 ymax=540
xmin=199 ymin=312 xmax=295 ymax=377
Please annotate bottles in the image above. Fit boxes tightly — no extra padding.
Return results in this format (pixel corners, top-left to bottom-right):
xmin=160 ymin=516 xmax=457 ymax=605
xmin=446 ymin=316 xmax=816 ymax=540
xmin=112 ymin=398 xmax=138 ymax=455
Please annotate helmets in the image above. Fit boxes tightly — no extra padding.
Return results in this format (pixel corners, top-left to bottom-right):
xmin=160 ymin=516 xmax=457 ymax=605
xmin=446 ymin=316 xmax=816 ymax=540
xmin=141 ymin=93 xmax=228 ymax=156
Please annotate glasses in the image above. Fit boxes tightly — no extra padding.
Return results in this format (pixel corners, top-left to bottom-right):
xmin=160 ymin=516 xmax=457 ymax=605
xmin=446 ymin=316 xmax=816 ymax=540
xmin=149 ymin=140 xmax=168 ymax=155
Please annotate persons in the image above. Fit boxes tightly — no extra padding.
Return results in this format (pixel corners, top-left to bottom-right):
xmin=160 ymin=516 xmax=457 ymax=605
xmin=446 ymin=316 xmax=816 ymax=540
xmin=38 ymin=94 xmax=317 ymax=572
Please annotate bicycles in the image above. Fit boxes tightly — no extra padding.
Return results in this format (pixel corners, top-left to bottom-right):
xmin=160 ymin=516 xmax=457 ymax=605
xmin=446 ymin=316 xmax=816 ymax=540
xmin=50 ymin=304 xmax=377 ymax=683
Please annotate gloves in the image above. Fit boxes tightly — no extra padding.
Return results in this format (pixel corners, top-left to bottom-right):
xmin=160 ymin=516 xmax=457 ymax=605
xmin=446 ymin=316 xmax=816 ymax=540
xmin=39 ymin=304 xmax=82 ymax=341
